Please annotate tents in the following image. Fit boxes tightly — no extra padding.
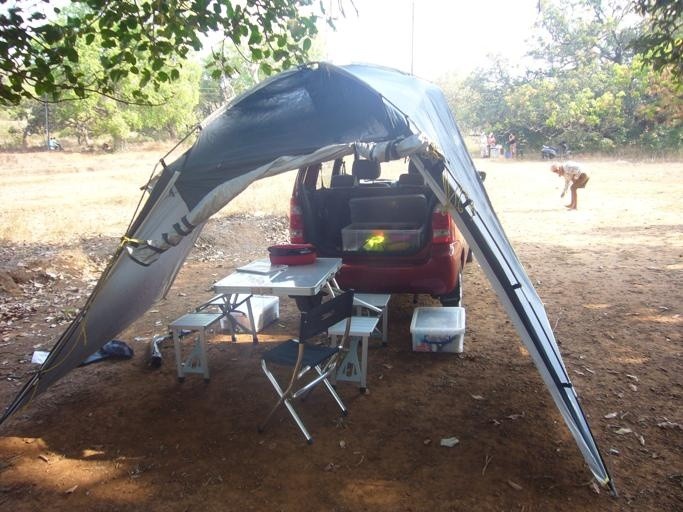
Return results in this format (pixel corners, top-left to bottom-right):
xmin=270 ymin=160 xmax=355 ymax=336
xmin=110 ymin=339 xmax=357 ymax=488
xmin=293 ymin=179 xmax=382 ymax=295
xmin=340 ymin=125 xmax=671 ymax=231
xmin=0 ymin=61 xmax=621 ymax=500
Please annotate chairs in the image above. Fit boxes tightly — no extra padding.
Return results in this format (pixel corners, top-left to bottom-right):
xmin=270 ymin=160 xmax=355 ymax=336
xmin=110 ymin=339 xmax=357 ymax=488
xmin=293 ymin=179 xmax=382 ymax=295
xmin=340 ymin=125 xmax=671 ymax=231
xmin=261 ymin=288 xmax=354 ymax=441
xmin=317 ymin=156 xmax=432 ymax=245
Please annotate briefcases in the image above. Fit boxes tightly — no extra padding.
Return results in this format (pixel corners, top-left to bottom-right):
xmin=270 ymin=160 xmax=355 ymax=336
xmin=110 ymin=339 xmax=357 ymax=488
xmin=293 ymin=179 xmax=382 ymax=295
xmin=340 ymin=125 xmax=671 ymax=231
xmin=268 ymin=244 xmax=317 ymax=265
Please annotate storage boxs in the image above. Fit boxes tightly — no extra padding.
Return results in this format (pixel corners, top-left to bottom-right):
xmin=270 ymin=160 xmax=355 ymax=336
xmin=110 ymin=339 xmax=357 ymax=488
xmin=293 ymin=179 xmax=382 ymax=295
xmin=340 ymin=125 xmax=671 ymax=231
xmin=217 ymin=296 xmax=280 ymax=335
xmin=339 ymin=222 xmax=424 ymax=254
xmin=410 ymin=306 xmax=465 ymax=353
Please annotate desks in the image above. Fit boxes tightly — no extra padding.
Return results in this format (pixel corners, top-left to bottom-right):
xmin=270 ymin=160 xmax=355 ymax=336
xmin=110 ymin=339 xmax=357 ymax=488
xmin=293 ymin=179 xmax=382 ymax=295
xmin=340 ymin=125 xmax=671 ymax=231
xmin=211 ymin=256 xmax=343 ymax=296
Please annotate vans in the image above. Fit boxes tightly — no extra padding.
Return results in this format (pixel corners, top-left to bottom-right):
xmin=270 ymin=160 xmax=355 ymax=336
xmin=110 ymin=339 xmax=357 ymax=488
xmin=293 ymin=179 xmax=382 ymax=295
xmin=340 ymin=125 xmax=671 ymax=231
xmin=290 ymin=153 xmax=487 ymax=311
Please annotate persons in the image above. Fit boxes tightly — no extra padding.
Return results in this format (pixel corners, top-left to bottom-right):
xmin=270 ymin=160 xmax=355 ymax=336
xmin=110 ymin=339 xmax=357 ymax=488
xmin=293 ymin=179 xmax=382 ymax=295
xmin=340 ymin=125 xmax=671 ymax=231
xmin=49 ymin=137 xmax=61 ymax=151
xmin=480 ymin=132 xmax=526 ymax=158
xmin=551 ymin=164 xmax=589 ymax=208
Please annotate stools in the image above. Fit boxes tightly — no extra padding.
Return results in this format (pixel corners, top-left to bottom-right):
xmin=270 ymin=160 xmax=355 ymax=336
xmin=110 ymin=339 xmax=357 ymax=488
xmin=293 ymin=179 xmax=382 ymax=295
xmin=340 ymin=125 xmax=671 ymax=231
xmin=166 ymin=294 xmax=258 ymax=384
xmin=326 ymin=291 xmax=390 ymax=397
